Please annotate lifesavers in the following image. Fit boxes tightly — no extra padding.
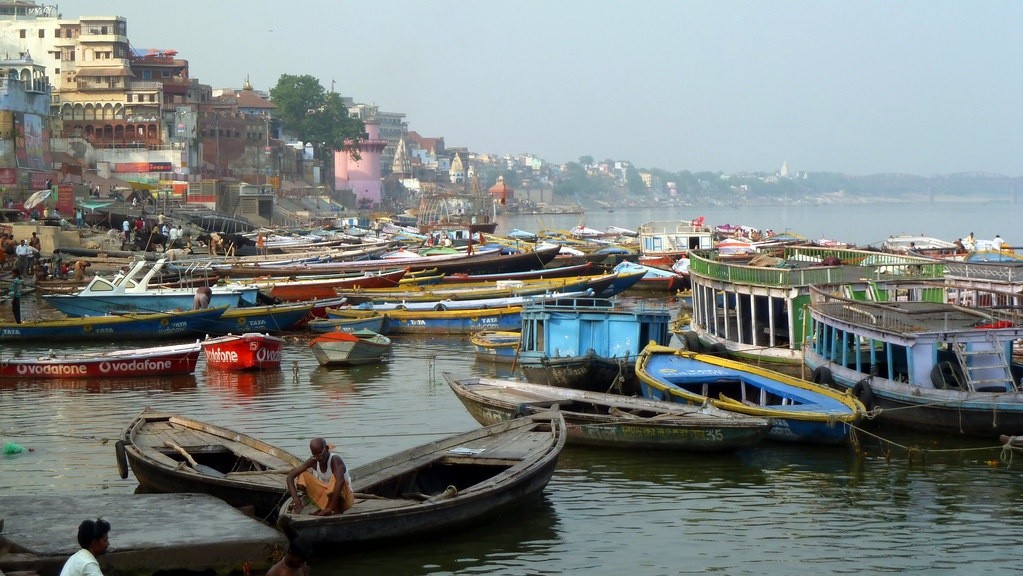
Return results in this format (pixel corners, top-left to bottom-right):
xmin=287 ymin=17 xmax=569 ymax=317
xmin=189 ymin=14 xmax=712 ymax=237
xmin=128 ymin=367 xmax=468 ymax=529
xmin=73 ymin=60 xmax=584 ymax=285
xmin=257 ymin=291 xmax=277 ymax=305
xmin=428 ymin=238 xmax=434 ymax=248
xmin=931 ymin=361 xmax=971 ymax=392
xmin=686 ymin=330 xmax=700 ymax=353
xmin=852 ymin=379 xmax=873 ymax=413
xmin=811 ymin=365 xmax=831 ymax=387
xmin=976 ymin=386 xmax=1008 ymax=393
xmin=710 ymin=342 xmax=727 ymax=359
xmin=975 ymin=320 xmax=1017 ymax=330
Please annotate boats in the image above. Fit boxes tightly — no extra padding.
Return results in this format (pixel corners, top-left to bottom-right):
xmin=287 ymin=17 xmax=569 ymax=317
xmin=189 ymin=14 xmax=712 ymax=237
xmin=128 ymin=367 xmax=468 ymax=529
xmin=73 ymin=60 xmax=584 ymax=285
xmin=307 ymin=323 xmax=392 ymax=368
xmin=516 ymin=347 xmax=640 ymax=395
xmin=0 ymin=193 xmax=1023 ymax=376
xmin=801 ymin=281 xmax=1023 ymax=438
xmin=440 ymin=371 xmax=781 ymax=456
xmin=117 ymin=412 xmax=315 ymax=510
xmin=201 ymin=331 xmax=287 ymax=372
xmin=276 ymin=405 xmax=568 ymax=548
xmin=0 ymin=338 xmax=202 ymax=376
xmin=633 ymin=339 xmax=877 ymax=448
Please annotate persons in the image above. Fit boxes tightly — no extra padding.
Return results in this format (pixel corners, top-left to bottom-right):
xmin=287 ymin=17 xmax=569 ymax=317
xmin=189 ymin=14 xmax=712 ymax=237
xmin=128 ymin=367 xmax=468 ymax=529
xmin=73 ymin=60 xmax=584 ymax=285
xmin=992 ymin=234 xmax=1006 ymax=253
xmin=122 ymin=212 xmax=184 ymax=250
xmin=0 ymin=232 xmax=91 ymax=280
xmin=965 ymin=232 xmax=977 ymax=253
xmin=46 ymin=178 xmax=138 ymax=208
xmin=909 ymin=241 xmax=918 ymax=256
xmin=428 ymin=233 xmax=452 ymax=250
xmin=734 ymin=227 xmax=774 ymax=242
xmin=58 ymin=517 xmax=115 ymax=576
xmin=210 ymin=230 xmax=237 ymax=258
xmin=953 ymin=238 xmax=965 ymax=254
xmin=193 ymin=286 xmax=212 ymax=310
xmin=107 ymin=227 xmax=117 ymax=246
xmin=286 ymin=436 xmax=354 ymax=516
xmin=8 ymin=198 xmax=88 ymax=231
xmin=374 ymin=218 xmax=380 ymax=238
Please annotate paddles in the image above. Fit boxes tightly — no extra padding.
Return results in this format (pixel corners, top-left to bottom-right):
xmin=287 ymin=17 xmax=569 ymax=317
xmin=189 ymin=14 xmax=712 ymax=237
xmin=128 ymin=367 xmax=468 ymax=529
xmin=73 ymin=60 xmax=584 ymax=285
xmin=351 ymin=494 xmax=395 ymax=500
xmin=226 ymin=469 xmax=292 ymax=475
xmin=54 ymin=351 xmax=136 ymax=359
xmin=162 ymin=440 xmax=227 ymax=477
xmin=401 ymin=490 xmax=437 ymax=500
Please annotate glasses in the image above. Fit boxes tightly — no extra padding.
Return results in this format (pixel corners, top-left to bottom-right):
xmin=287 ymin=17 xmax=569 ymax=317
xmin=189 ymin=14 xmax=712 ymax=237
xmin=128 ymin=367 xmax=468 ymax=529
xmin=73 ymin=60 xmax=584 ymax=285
xmin=313 ymin=447 xmax=326 ymax=459
